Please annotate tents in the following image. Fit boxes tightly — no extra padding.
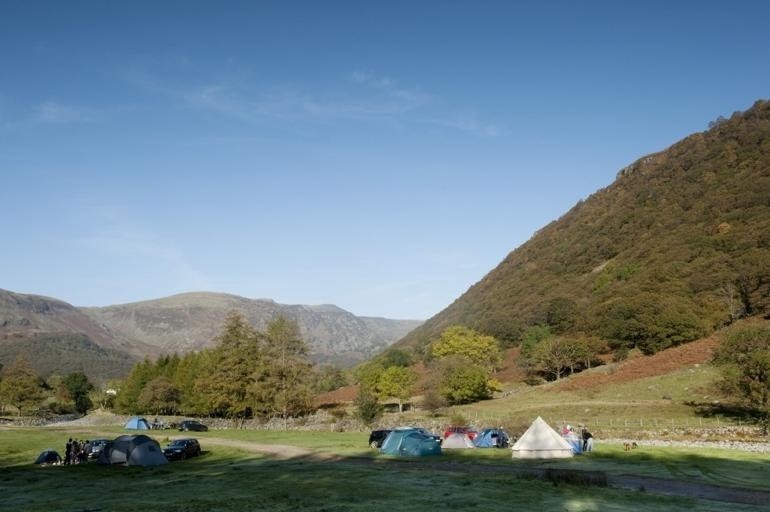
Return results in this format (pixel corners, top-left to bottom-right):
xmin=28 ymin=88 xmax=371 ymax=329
xmin=34 ymin=449 xmax=62 ymax=464
xmin=511 ymin=416 xmax=574 ymax=459
xmin=472 ymin=427 xmax=510 ymax=448
xmin=378 ymin=426 xmax=443 ymax=456
xmin=440 ymin=428 xmax=475 ymax=449
xmin=123 ymin=416 xmax=151 ymax=430
xmin=91 ymin=436 xmax=169 ymax=466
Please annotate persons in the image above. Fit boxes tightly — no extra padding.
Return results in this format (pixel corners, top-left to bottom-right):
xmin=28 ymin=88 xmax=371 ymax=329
xmin=150 ymin=417 xmax=177 ymax=430
xmin=63 ymin=438 xmax=90 ymax=464
xmin=582 ymin=423 xmax=594 ymax=452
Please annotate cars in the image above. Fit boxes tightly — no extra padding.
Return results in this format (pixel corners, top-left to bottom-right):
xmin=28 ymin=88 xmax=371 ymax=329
xmin=84 ymin=438 xmax=110 ymax=459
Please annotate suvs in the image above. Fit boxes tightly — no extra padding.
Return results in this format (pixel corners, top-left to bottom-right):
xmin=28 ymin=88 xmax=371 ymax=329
xmin=444 ymin=425 xmax=478 ymax=443
xmin=413 ymin=427 xmax=442 ymax=446
xmin=367 ymin=428 xmax=395 ymax=449
xmin=160 ymin=438 xmax=202 ymax=462
xmin=176 ymin=420 xmax=208 ymax=432
